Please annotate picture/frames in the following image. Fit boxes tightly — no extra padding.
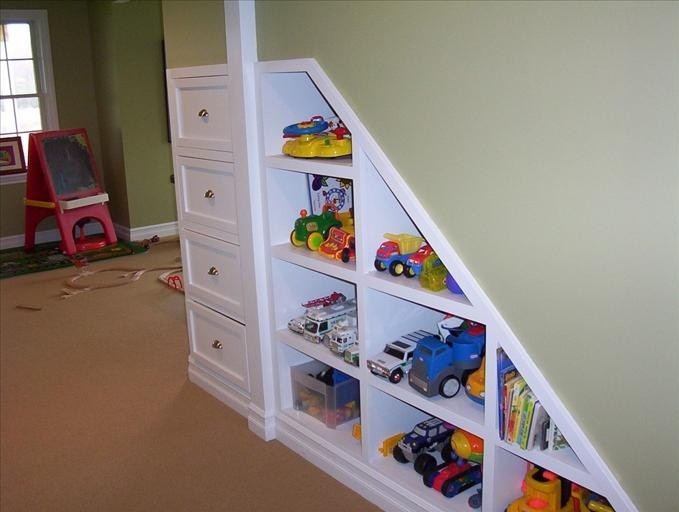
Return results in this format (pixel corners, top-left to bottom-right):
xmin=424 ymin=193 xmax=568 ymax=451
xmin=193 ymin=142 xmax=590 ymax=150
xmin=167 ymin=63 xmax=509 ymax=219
xmin=0 ymin=136 xmax=27 ymax=176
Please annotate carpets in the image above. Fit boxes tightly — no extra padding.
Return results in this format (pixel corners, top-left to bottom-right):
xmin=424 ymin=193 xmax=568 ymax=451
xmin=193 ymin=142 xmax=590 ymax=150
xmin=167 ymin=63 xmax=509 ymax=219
xmin=0 ymin=231 xmax=149 ymax=281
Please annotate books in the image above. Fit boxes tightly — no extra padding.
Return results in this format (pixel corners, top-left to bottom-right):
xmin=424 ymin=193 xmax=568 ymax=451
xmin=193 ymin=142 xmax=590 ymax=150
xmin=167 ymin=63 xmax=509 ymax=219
xmin=497 ymin=348 xmax=569 ymax=451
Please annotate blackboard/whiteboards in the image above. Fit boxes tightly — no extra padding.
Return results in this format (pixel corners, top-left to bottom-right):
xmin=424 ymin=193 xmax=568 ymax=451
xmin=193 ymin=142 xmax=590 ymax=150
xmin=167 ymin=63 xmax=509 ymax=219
xmin=33 ymin=127 xmax=102 ymax=202
